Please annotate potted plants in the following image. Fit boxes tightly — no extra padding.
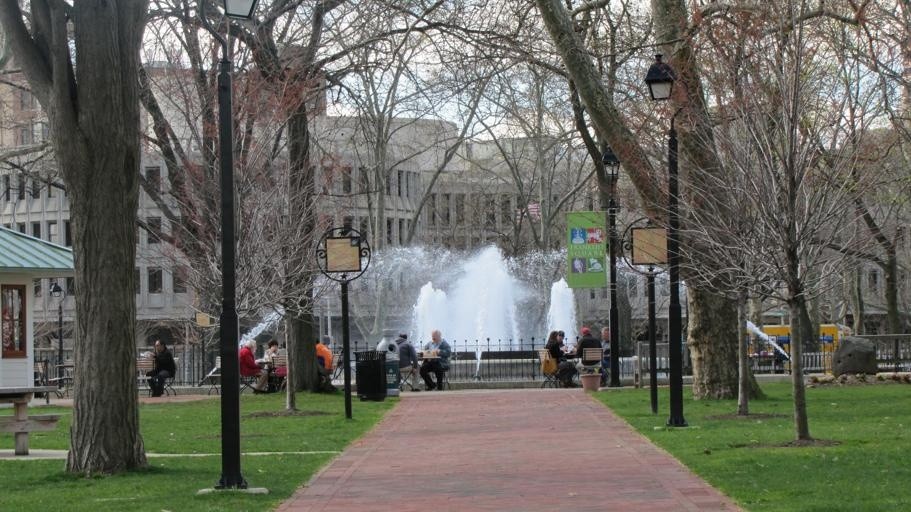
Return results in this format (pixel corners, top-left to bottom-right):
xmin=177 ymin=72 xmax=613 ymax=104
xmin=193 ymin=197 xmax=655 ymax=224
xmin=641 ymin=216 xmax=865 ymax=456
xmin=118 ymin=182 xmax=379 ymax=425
xmin=579 ymin=368 xmax=604 ymax=392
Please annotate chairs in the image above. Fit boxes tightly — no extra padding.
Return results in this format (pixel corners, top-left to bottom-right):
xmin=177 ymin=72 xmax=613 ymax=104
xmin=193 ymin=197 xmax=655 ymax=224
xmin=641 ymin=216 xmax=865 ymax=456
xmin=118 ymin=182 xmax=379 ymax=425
xmin=34 ymin=358 xmax=73 ymax=398
xmin=328 ymin=347 xmax=346 ymax=381
xmin=398 ymin=350 xmax=457 ymax=392
xmin=206 ymin=355 xmax=222 ymax=396
xmin=241 ymin=354 xmax=287 ymax=394
xmin=534 ymin=344 xmax=624 ymax=388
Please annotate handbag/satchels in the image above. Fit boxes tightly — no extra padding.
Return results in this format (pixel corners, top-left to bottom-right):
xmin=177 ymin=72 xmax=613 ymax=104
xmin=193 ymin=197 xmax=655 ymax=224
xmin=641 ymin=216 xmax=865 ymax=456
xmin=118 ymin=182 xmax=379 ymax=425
xmin=542 ymin=350 xmax=557 ymax=373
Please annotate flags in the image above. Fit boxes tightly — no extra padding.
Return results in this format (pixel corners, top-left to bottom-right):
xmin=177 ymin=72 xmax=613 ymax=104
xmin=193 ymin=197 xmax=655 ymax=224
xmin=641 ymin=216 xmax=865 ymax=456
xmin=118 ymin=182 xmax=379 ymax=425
xmin=517 ymin=203 xmax=542 ymax=227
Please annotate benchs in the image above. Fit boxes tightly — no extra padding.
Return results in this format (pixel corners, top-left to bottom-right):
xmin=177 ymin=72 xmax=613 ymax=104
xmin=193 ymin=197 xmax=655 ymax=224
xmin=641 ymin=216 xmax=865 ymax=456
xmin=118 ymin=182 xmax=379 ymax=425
xmin=134 ymin=357 xmax=181 ymax=397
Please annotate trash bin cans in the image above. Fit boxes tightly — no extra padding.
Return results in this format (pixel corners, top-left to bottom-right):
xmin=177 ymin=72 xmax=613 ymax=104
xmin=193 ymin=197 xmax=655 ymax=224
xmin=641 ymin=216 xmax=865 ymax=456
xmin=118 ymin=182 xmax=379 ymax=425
xmin=353 ymin=351 xmax=387 ymax=402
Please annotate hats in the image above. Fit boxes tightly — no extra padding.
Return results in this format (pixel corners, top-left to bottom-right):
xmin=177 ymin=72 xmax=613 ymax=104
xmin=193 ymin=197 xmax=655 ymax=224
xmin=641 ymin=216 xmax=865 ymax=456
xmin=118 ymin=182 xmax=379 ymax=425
xmin=582 ymin=328 xmax=589 ymax=334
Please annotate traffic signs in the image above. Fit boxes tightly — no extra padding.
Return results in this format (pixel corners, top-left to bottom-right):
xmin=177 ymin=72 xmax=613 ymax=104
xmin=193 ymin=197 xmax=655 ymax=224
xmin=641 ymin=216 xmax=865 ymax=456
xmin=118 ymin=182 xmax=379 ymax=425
xmin=603 ymin=147 xmax=622 ymax=387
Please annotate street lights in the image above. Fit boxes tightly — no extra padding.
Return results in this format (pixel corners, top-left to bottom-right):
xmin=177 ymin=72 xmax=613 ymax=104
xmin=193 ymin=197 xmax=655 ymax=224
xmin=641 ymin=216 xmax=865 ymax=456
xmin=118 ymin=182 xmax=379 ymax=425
xmin=195 ymin=0 xmax=271 ymax=497
xmin=645 ymin=53 xmax=688 ymax=428
xmin=50 ymin=282 xmax=66 ymax=390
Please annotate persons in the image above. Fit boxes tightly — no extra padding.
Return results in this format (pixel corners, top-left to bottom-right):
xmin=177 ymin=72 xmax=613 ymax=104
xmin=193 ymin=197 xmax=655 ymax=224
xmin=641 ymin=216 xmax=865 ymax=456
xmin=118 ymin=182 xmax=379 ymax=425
xmin=264 ymin=339 xmax=280 ymax=394
xmin=276 ymin=341 xmax=285 ymax=392
xmin=574 ymin=326 xmax=607 ymax=389
xmin=545 ymin=330 xmax=578 ymax=388
xmin=146 ymin=339 xmax=177 ymax=397
xmin=389 ymin=332 xmax=421 ymax=392
xmin=239 ymin=340 xmax=269 ymax=394
xmin=419 ymin=330 xmax=451 ymax=391
xmin=599 ymin=327 xmax=612 ymax=387
xmin=316 ymin=337 xmax=332 ymax=391
